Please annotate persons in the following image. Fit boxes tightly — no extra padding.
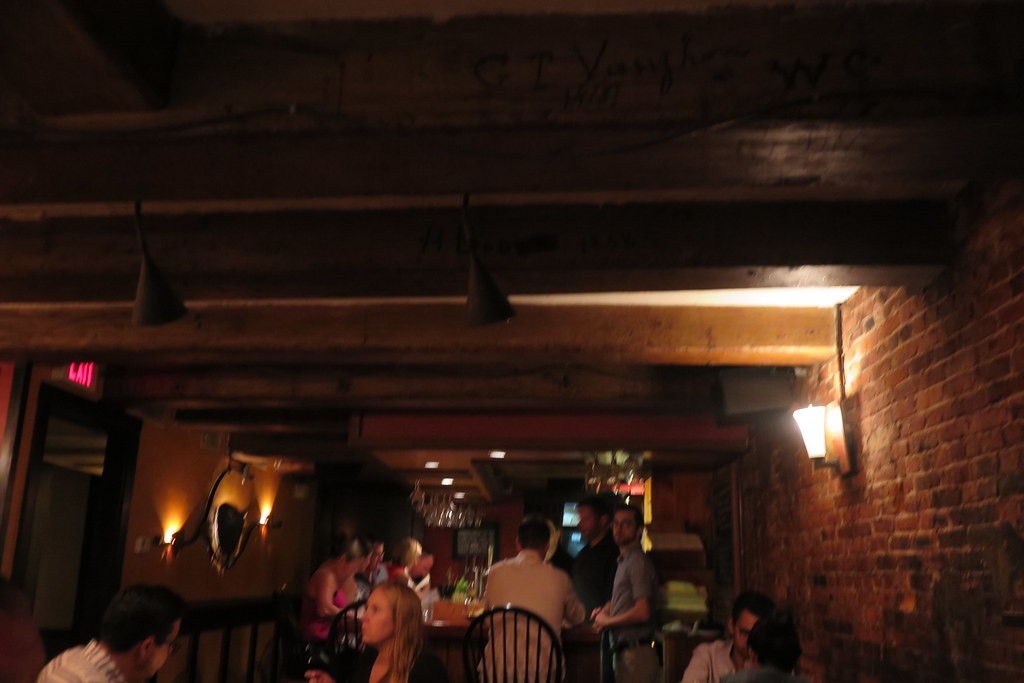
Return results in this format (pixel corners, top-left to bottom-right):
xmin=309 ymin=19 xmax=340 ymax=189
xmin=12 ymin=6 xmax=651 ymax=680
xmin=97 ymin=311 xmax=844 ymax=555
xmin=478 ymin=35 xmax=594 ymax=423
xmin=387 ymin=535 xmax=434 ymax=601
xmin=569 ymin=492 xmax=620 ymax=606
xmin=523 ymin=515 xmax=573 ymax=578
xmin=680 ymin=589 xmax=779 ymax=683
xmin=590 ymin=506 xmax=660 ymax=683
xmin=305 ymin=579 xmax=450 ymax=683
xmin=36 ymin=584 xmax=187 ymax=683
xmin=719 ymin=616 xmax=810 ymax=683
xmin=476 ymin=517 xmax=585 ymax=683
xmin=301 ymin=531 xmax=388 ymax=653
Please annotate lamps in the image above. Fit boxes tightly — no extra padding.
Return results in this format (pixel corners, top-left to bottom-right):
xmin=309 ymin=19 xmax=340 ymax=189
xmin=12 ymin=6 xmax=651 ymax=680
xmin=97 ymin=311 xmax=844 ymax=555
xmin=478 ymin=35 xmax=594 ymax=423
xmin=794 ymin=402 xmax=850 ymax=480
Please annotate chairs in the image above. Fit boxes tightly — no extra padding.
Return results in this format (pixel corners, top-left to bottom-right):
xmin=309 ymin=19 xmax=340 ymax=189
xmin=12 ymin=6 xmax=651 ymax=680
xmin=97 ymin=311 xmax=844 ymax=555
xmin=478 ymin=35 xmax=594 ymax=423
xmin=599 ymin=622 xmax=670 ymax=683
xmin=328 ymin=599 xmax=366 ymax=655
xmin=462 ymin=606 xmax=561 ymax=683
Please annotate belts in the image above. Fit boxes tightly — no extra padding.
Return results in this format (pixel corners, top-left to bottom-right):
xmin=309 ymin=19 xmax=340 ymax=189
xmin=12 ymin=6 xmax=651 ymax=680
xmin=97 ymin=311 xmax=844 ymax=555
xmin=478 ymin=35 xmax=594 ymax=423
xmin=614 ymin=638 xmax=649 ymax=651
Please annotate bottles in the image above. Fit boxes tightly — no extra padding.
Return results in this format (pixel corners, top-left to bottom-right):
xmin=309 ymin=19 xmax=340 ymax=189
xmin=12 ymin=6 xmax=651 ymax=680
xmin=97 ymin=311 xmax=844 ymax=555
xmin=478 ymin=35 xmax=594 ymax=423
xmin=442 ymin=580 xmax=452 ymax=600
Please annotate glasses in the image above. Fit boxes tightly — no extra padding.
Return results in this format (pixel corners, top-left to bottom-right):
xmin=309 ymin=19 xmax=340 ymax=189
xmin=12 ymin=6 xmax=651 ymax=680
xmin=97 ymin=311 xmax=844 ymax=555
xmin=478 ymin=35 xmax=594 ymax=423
xmin=736 ymin=623 xmax=750 ymax=639
xmin=163 ymin=640 xmax=182 ymax=655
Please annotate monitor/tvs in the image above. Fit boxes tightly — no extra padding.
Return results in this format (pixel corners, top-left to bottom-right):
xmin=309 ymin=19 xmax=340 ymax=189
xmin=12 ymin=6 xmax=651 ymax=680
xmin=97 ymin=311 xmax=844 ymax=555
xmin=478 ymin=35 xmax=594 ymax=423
xmin=562 ymin=502 xmax=581 ymax=527
